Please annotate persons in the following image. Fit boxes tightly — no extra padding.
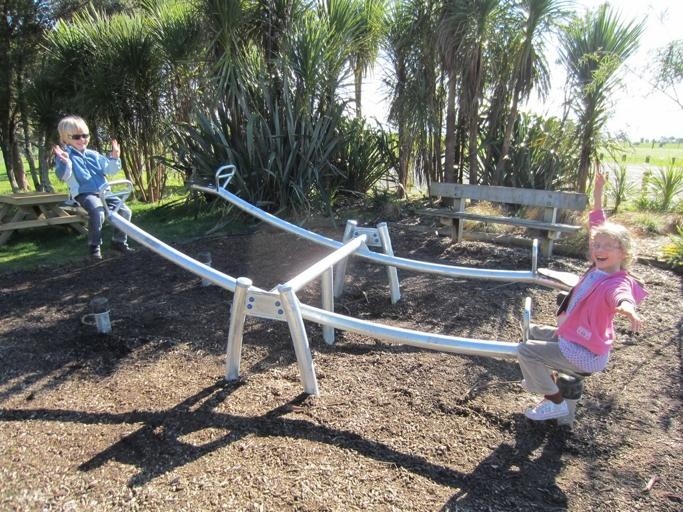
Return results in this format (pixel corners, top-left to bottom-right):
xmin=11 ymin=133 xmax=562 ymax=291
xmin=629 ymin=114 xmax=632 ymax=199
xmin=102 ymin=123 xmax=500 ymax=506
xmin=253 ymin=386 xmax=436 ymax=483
xmin=51 ymin=114 xmax=136 ymax=262
xmin=517 ymin=170 xmax=641 ymax=420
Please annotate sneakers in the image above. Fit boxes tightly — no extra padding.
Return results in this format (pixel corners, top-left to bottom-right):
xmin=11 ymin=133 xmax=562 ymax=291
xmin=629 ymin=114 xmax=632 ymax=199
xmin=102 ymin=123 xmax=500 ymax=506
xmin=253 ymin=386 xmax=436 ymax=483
xmin=520 ymin=378 xmax=532 ymax=393
xmin=111 ymin=239 xmax=135 ymax=254
xmin=525 ymin=396 xmax=570 ymax=421
xmin=87 ymin=243 xmax=103 ymax=260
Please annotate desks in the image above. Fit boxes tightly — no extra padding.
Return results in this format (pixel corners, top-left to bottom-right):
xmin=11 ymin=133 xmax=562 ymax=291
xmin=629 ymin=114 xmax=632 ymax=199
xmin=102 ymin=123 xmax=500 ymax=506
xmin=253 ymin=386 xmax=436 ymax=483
xmin=0 ymin=192 xmax=89 ymax=245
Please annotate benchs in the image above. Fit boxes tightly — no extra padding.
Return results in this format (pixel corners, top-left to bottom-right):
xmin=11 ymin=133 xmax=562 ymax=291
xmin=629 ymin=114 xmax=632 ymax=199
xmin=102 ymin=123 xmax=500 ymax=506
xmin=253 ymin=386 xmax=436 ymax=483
xmin=0 ymin=211 xmax=82 ymax=233
xmin=413 ymin=181 xmax=588 ymax=258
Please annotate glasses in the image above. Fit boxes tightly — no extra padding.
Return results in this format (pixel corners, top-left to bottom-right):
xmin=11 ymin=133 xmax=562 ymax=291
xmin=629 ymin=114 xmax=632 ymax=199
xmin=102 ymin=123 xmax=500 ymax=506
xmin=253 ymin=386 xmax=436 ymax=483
xmin=67 ymin=134 xmax=89 ymax=140
xmin=589 ymin=241 xmax=622 ymax=252
